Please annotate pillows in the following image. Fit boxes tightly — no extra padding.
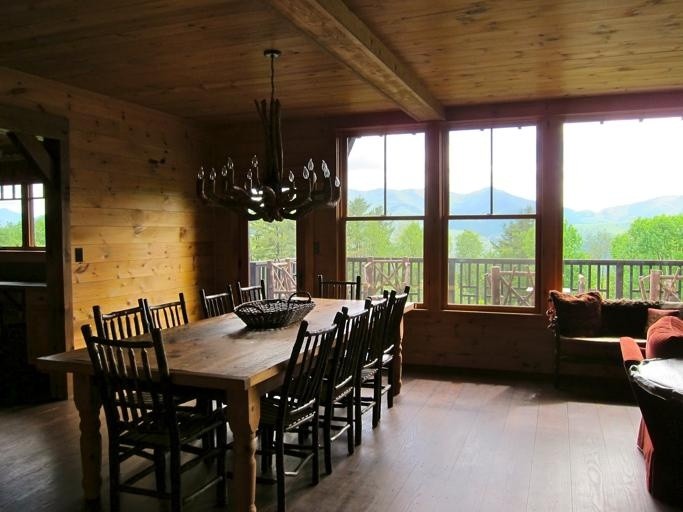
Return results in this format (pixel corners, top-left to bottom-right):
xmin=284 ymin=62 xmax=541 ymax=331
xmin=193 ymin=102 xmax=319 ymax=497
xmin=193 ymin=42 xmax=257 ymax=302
xmin=644 ymin=307 xmax=683 ymax=339
xmin=550 ymin=288 xmax=604 ymax=337
xmin=645 ymin=314 xmax=683 ymax=359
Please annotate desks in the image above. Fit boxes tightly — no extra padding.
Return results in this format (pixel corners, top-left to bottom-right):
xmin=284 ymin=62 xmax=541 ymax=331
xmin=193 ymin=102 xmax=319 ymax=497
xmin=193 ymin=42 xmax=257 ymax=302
xmin=38 ymin=297 xmax=415 ymax=511
xmin=626 ymin=359 xmax=683 ymax=509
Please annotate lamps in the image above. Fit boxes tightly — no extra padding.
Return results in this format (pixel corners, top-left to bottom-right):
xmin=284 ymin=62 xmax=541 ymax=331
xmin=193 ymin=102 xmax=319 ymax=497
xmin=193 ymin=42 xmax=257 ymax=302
xmin=195 ymin=48 xmax=342 ymax=222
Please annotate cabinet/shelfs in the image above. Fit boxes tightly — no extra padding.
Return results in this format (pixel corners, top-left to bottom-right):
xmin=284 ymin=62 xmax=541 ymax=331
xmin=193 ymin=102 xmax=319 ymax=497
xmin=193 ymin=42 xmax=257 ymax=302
xmin=24 ymin=286 xmax=50 ymax=364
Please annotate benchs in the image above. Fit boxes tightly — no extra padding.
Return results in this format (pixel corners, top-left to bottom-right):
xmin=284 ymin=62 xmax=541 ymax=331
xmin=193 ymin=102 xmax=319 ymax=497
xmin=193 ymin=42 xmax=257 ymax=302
xmin=552 ymin=300 xmax=683 ymax=390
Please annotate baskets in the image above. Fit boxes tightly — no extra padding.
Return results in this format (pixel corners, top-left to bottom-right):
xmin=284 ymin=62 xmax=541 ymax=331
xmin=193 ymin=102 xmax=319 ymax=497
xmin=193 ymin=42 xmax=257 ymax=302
xmin=233 ymin=292 xmax=316 ymax=329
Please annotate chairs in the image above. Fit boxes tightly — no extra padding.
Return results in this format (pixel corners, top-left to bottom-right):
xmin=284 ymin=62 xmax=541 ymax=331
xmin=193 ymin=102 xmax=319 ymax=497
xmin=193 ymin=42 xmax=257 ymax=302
xmin=268 ymin=299 xmax=370 ymax=476
xmin=201 ymin=284 xmax=236 ymax=319
xmin=368 ymin=286 xmax=412 ymax=420
xmin=319 ymin=290 xmax=386 ymax=445
xmin=91 ymin=299 xmax=206 ymax=462
xmin=214 ymin=312 xmax=343 ymax=511
xmin=238 ymin=280 xmax=266 ymax=306
xmin=619 ymin=336 xmax=653 ymax=496
xmin=144 ymin=292 xmax=209 ymax=414
xmin=80 ymin=323 xmax=228 ymax=511
